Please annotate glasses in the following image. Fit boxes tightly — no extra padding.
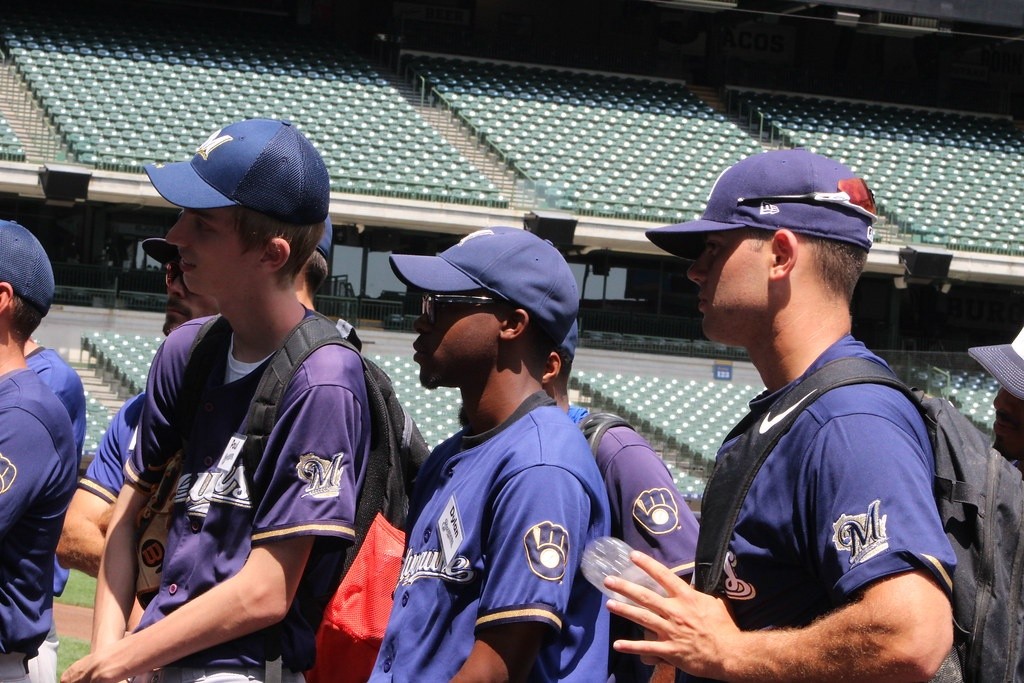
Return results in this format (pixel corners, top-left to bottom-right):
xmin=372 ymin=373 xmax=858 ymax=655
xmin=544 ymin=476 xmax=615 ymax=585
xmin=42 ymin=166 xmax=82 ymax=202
xmin=737 ymin=178 xmax=878 ymax=224
xmin=421 ymin=290 xmax=512 ymax=326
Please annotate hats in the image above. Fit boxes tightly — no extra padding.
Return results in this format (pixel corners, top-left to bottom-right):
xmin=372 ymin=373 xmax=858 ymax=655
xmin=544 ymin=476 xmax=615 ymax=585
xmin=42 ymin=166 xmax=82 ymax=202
xmin=558 ymin=316 xmax=579 ymax=360
xmin=317 ymin=213 xmax=332 ymax=257
xmin=143 ymin=118 xmax=330 ymax=226
xmin=0 ymin=219 xmax=54 ymax=317
xmin=389 ymin=226 xmax=579 ymax=332
xmin=645 ymin=146 xmax=878 ymax=261
xmin=142 ymin=209 xmax=186 ymax=266
xmin=968 ymin=327 xmax=1024 ymax=400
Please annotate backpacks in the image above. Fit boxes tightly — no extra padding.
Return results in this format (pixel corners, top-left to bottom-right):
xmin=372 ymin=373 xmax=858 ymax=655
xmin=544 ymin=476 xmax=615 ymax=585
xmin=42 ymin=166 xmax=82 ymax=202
xmin=676 ymin=357 xmax=1024 ymax=683
xmin=181 ymin=312 xmax=431 ymax=683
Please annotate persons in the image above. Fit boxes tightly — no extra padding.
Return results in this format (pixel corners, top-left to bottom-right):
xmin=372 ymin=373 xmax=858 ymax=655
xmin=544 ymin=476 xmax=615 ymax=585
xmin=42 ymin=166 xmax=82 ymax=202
xmin=0 ymin=218 xmax=78 ymax=683
xmin=604 ymin=149 xmax=957 ymax=683
xmin=969 ymin=325 xmax=1024 ymax=480
xmin=55 ymin=235 xmax=223 ymax=579
xmin=62 ymin=116 xmax=372 ymax=682
xmin=370 ymin=227 xmax=612 ymax=683
xmin=541 ymin=318 xmax=698 ymax=683
xmin=23 ymin=338 xmax=86 ymax=597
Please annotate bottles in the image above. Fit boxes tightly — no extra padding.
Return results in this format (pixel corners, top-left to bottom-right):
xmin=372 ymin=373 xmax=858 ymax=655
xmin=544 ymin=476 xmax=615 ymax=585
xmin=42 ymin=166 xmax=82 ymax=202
xmin=580 ymin=536 xmax=668 ymax=617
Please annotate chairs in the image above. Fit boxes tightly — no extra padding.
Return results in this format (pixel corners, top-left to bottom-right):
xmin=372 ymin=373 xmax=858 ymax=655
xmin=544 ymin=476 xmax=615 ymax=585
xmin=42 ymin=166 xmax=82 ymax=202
xmin=29 ymin=288 xmax=170 ymax=455
xmin=901 ymin=363 xmax=1002 ymax=440
xmin=569 ymin=329 xmax=769 ymax=508
xmin=0 ymin=10 xmax=1024 ymax=257
xmin=361 ymin=314 xmax=465 ymax=454
